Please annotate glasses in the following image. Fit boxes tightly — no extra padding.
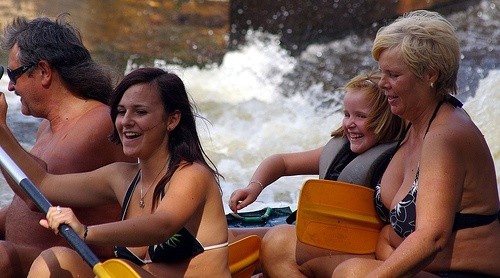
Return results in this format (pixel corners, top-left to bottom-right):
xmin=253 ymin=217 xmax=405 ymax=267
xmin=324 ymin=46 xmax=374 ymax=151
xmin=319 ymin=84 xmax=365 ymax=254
xmin=7 ymin=58 xmax=39 ymax=85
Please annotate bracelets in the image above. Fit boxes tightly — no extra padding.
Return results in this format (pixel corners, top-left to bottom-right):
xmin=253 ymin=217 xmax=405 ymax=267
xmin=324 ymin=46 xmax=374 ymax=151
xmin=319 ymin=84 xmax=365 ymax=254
xmin=247 ymin=180 xmax=263 ymax=190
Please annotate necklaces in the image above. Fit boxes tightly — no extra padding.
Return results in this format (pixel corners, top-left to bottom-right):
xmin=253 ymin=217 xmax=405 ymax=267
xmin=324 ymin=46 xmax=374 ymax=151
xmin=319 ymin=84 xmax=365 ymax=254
xmin=139 ymin=157 xmax=170 ymax=208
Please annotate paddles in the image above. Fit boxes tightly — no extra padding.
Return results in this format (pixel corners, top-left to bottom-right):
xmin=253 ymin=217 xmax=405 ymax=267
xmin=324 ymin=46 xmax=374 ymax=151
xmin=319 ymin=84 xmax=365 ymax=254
xmin=0 ymin=146 xmax=142 ymax=278
xmin=226 ymin=236 xmax=261 ymax=278
xmin=294 ymin=179 xmax=386 ymax=255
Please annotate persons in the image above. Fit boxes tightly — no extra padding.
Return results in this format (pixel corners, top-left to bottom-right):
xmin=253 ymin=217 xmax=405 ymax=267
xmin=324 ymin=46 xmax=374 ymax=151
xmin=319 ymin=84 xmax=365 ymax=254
xmin=229 ymin=75 xmax=409 ymax=278
xmin=0 ymin=68 xmax=229 ymax=278
xmin=0 ymin=11 xmax=138 ymax=278
xmin=260 ymin=10 xmax=500 ymax=278
